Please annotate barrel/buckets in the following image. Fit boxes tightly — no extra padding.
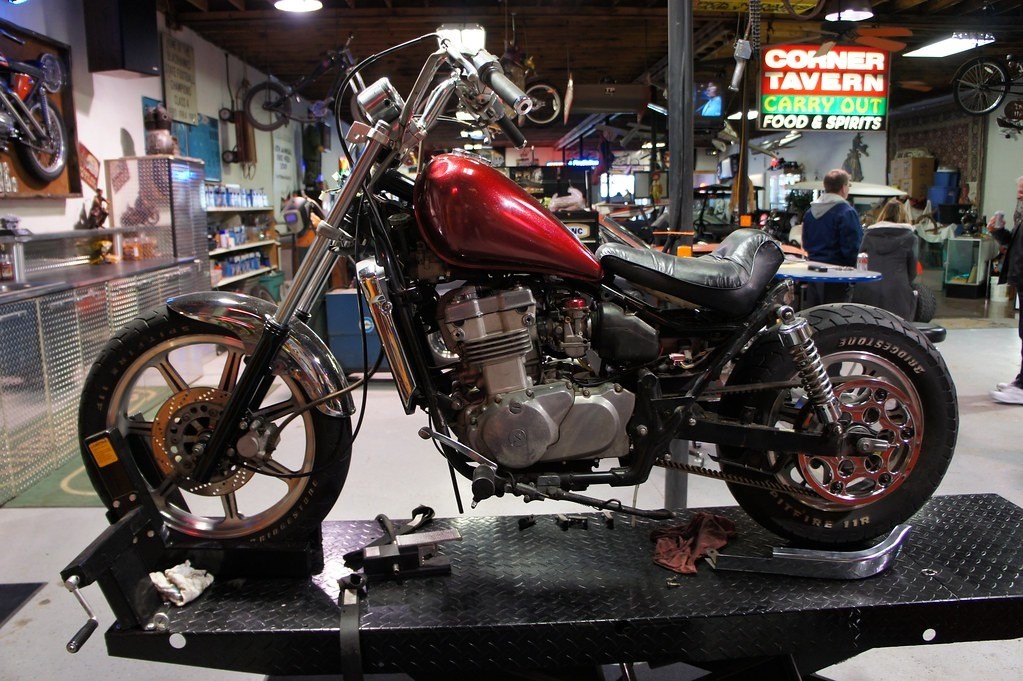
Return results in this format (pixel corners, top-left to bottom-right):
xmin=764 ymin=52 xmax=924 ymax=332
xmin=990 ymin=277 xmax=1010 ymax=302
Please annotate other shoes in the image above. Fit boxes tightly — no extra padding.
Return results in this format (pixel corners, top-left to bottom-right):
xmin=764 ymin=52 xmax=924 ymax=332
xmin=996 ymin=382 xmax=1017 ymax=392
xmin=990 ymin=386 xmax=1023 ymax=404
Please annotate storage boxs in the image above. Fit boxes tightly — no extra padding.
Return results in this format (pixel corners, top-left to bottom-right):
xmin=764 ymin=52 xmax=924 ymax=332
xmin=890 ymin=157 xmax=934 ymax=199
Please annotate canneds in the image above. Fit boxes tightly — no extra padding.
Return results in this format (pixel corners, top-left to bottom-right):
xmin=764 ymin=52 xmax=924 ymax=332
xmin=994 ymin=211 xmax=1004 ymax=228
xmin=856 ymin=252 xmax=868 ymax=272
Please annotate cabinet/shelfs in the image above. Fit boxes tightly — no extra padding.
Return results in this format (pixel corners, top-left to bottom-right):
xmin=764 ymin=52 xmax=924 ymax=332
xmin=1 ymin=154 xmax=206 ymax=506
xmin=204 ymin=207 xmax=283 ymax=288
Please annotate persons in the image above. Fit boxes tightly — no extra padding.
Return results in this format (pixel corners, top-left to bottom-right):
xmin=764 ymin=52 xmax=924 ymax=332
xmin=86 ymin=188 xmax=109 ymax=230
xmin=649 ymin=172 xmax=663 ymax=204
xmin=702 ymin=82 xmax=721 ymax=115
xmin=960 ymin=184 xmax=970 ymax=203
xmin=135 ymin=190 xmax=148 ymax=224
xmin=859 ymin=200 xmax=919 ymax=323
xmin=987 ymin=177 xmax=1023 ymax=405
xmin=803 ymin=170 xmax=864 ymax=305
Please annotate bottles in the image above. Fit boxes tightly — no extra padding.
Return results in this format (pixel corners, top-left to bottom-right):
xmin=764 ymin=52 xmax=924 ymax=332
xmin=205 ymin=190 xmax=267 ymax=207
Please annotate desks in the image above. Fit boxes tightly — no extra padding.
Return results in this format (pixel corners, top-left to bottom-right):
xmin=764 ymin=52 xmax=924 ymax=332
xmin=690 ymin=243 xmax=884 ymax=310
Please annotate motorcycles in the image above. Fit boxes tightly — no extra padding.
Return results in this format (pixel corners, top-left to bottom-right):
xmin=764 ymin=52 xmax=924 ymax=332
xmin=952 ymin=32 xmax=1019 ymax=142
xmin=0 ymin=30 xmax=73 ymax=182
xmin=77 ymin=29 xmax=964 ymax=558
xmin=243 ymin=35 xmax=365 ymax=134
